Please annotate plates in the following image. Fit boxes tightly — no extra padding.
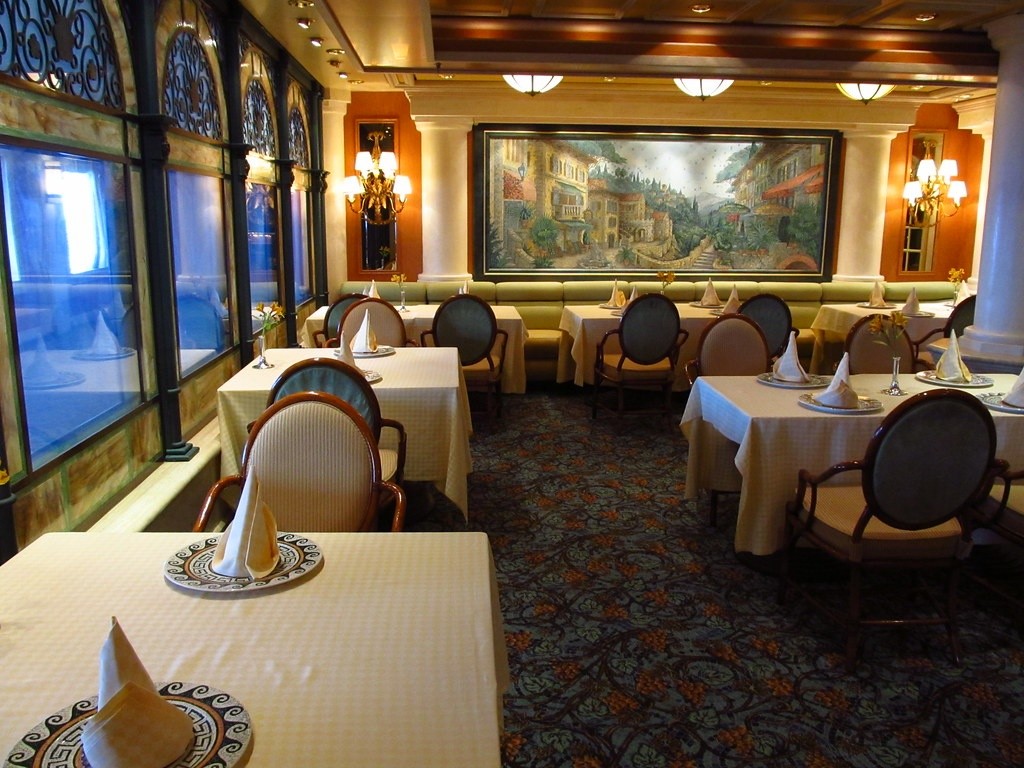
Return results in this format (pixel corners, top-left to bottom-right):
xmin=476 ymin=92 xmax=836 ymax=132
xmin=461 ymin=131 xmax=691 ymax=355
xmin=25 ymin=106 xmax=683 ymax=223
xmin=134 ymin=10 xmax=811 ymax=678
xmin=799 ymin=393 xmax=883 ymax=412
xmin=163 ymin=533 xmax=323 ymax=592
xmin=600 ymin=302 xmax=723 ymax=316
xmin=756 ymin=372 xmax=830 ymax=387
xmin=334 ymin=345 xmax=396 ymax=358
xmin=916 ymin=370 xmax=994 ymax=386
xmin=976 ymin=392 xmax=1024 ymax=414
xmin=363 ymin=370 xmax=382 ymax=382
xmin=3 ymin=681 xmax=251 ymax=768
xmin=858 ymin=302 xmax=897 ymax=308
xmin=899 ymin=310 xmax=935 ymax=316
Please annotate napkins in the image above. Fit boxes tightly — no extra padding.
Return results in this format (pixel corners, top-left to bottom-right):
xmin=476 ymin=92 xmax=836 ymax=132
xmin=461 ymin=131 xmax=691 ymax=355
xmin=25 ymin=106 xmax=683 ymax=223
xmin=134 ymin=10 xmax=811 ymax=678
xmin=628 ymin=283 xmax=638 ymax=305
xmin=721 ymin=283 xmax=741 ymax=315
xmin=772 ymin=331 xmax=810 ymax=381
xmin=336 ymin=330 xmax=364 ymax=377
xmin=80 ymin=616 xmax=194 ymax=767
xmin=348 ymin=308 xmax=378 ymax=353
xmin=699 ymin=277 xmax=720 ymax=306
xmin=207 ymin=464 xmax=281 ymax=579
xmin=900 ymin=287 xmax=921 ymax=317
xmin=811 ymin=351 xmax=862 ymax=408
xmin=933 ymin=328 xmax=973 ymax=382
xmin=868 ymin=280 xmax=885 ymax=306
xmin=605 ymin=278 xmax=620 ymax=307
xmin=367 ymin=279 xmax=382 ymax=299
xmin=1001 ymin=367 xmax=1024 ymax=409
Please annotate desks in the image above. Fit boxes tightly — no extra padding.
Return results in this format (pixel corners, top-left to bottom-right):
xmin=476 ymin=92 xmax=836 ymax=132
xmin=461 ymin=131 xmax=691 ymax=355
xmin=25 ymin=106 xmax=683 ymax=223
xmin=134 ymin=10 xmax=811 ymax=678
xmin=677 ymin=373 xmax=1024 ymax=556
xmin=1 ymin=531 xmax=509 ymax=768
xmin=299 ymin=303 xmax=528 ymax=395
xmin=217 ymin=347 xmax=476 ymax=524
xmin=559 ymin=302 xmax=725 ymax=389
xmin=810 ymin=301 xmax=961 ymax=373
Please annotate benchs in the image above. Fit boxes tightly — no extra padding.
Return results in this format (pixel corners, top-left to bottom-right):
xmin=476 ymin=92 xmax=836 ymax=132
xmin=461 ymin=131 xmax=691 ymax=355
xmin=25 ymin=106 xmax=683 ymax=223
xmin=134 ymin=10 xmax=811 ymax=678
xmin=329 ymin=279 xmax=960 ymax=382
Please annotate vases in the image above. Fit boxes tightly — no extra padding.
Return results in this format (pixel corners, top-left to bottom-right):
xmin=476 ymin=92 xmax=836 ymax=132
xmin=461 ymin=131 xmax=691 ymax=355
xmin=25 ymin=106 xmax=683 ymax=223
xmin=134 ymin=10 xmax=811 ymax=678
xmin=252 ymin=335 xmax=274 ymax=369
xmin=395 ymin=291 xmax=409 ymax=312
xmin=881 ymin=356 xmax=907 ymax=395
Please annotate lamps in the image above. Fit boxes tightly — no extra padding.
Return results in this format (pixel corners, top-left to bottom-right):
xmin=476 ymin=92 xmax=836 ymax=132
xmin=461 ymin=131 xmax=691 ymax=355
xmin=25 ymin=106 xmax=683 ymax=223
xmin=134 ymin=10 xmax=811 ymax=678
xmin=337 ymin=131 xmax=413 ymax=226
xmin=673 ymin=77 xmax=734 ymax=102
xmin=834 ymin=83 xmax=897 ymax=106
xmin=901 ymin=132 xmax=968 ymax=231
xmin=501 ymin=74 xmax=565 ymax=97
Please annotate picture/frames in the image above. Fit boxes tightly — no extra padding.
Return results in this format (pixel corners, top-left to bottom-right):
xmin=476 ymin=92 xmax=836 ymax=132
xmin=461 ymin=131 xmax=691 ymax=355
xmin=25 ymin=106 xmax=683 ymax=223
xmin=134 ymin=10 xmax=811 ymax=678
xmin=473 ymin=122 xmax=841 ymax=282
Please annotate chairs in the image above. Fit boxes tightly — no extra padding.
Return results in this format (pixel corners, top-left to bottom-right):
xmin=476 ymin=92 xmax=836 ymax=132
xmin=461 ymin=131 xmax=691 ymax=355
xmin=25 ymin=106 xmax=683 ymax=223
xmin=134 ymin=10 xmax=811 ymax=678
xmin=192 ymin=388 xmax=409 ymax=534
xmin=950 ymin=460 xmax=1024 ymax=635
xmin=309 ymin=292 xmax=975 ymax=421
xmin=245 ymin=357 xmax=404 ymax=510
xmin=776 ymin=388 xmax=1012 ymax=666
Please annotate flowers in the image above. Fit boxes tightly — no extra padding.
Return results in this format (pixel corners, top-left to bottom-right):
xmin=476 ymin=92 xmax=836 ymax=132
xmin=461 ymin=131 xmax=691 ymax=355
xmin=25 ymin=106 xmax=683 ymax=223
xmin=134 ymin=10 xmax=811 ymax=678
xmin=389 ymin=274 xmax=408 ymax=291
xmin=867 ymin=310 xmax=910 ymax=357
xmin=656 ymin=269 xmax=676 ymax=291
xmin=255 ymin=302 xmax=286 ymax=337
xmin=948 ymin=267 xmax=966 ymax=301
